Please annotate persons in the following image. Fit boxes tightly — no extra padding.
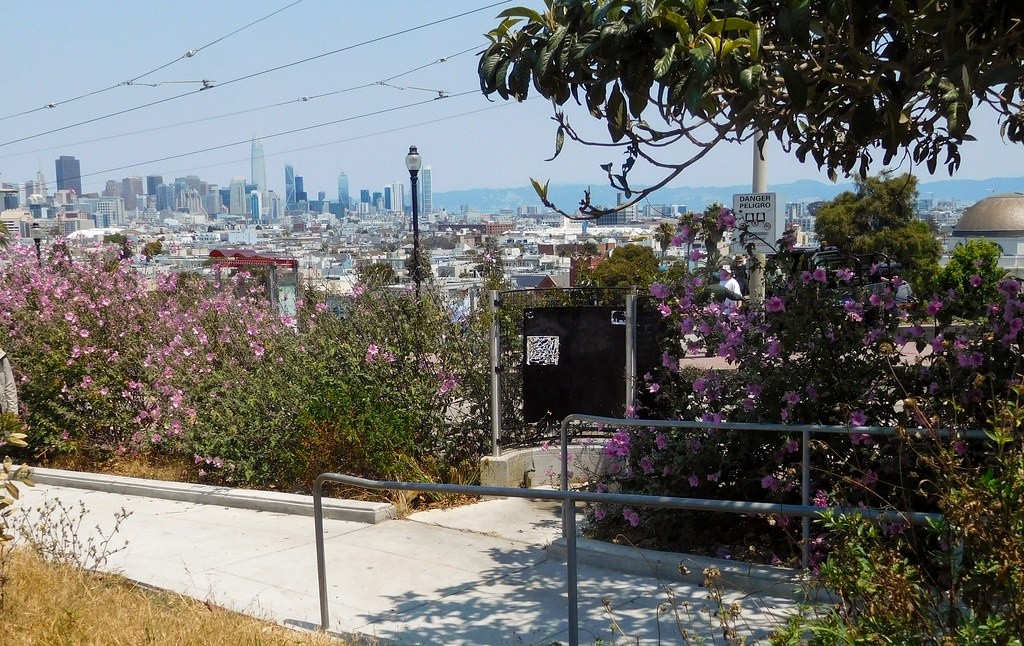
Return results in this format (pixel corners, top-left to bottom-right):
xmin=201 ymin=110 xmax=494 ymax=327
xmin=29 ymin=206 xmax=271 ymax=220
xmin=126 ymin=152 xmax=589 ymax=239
xmin=719 ymin=254 xmax=748 ymax=309
xmin=0 ymin=348 xmax=19 ymax=446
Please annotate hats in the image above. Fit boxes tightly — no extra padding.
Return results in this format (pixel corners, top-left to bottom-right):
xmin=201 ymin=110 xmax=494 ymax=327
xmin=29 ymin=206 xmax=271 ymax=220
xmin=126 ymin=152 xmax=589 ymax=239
xmin=717 ymin=264 xmax=732 ymax=274
xmin=735 ymin=254 xmax=744 ymax=261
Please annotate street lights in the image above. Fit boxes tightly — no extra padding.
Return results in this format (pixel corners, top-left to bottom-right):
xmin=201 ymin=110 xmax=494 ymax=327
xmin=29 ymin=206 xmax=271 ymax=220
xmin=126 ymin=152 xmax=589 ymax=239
xmin=404 ymin=144 xmax=423 ymax=371
